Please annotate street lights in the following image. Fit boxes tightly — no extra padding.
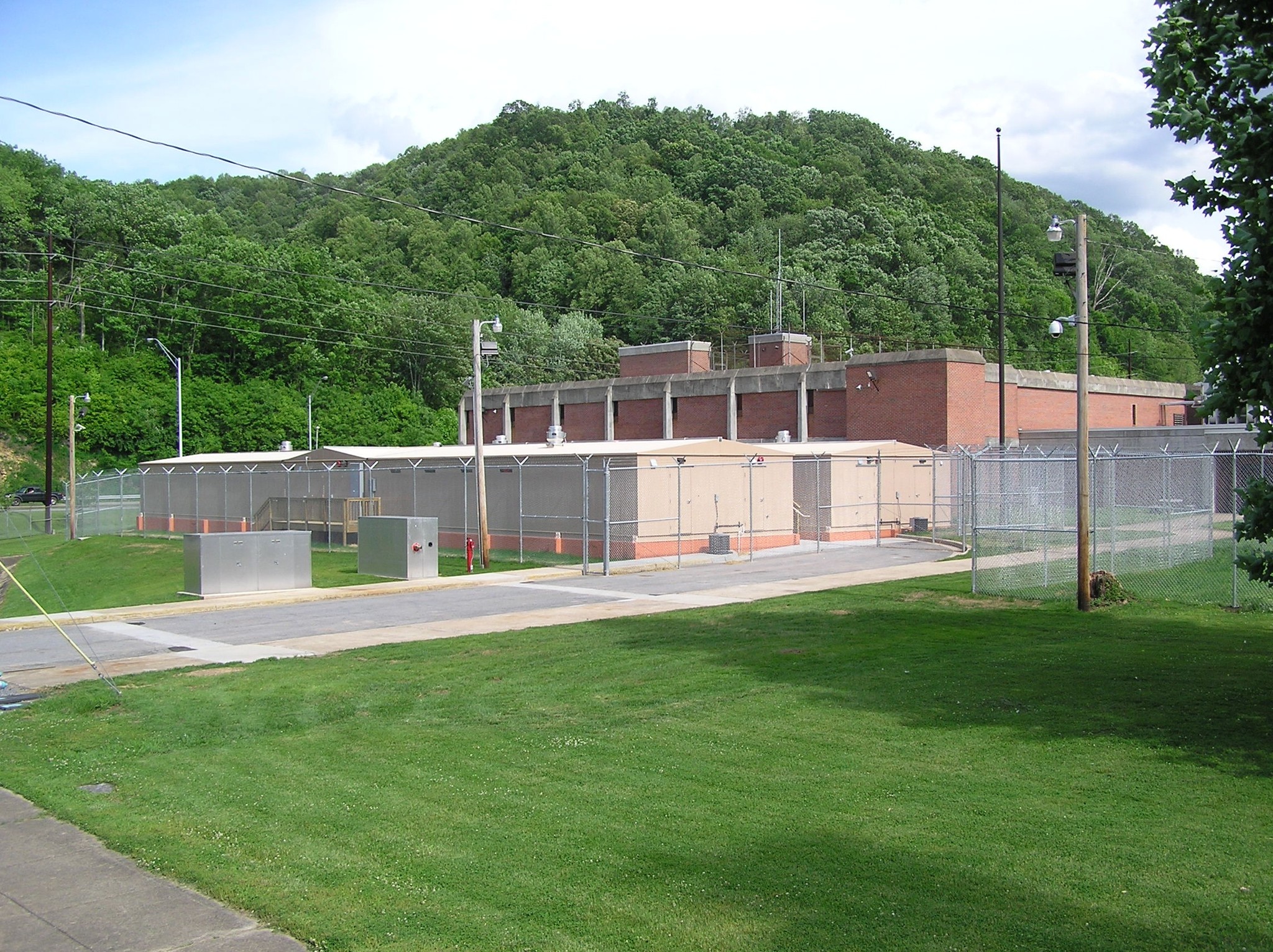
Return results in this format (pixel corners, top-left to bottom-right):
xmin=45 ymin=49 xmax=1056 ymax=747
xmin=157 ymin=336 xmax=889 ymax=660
xmin=308 ymin=375 xmax=328 ymax=450
xmin=70 ymin=392 xmax=91 ymax=538
xmin=1045 ymin=213 xmax=1091 ymax=611
xmin=147 ymin=337 xmax=183 ymax=457
xmin=472 ymin=317 xmax=503 ymax=565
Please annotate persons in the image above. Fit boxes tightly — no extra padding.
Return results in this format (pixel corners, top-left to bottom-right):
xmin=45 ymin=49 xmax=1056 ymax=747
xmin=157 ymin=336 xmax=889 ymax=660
xmin=26 ymin=489 xmax=34 ymax=494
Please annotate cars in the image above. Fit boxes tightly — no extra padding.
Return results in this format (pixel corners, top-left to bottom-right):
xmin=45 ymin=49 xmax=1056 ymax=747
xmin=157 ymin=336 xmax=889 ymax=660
xmin=4 ymin=486 xmax=64 ymax=505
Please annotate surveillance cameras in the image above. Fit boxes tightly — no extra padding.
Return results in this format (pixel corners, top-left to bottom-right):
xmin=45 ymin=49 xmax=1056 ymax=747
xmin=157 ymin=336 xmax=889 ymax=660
xmin=76 ymin=424 xmax=87 ymax=431
xmin=856 ymin=384 xmax=862 ymax=390
xmin=1049 ymin=321 xmax=1063 ymax=338
